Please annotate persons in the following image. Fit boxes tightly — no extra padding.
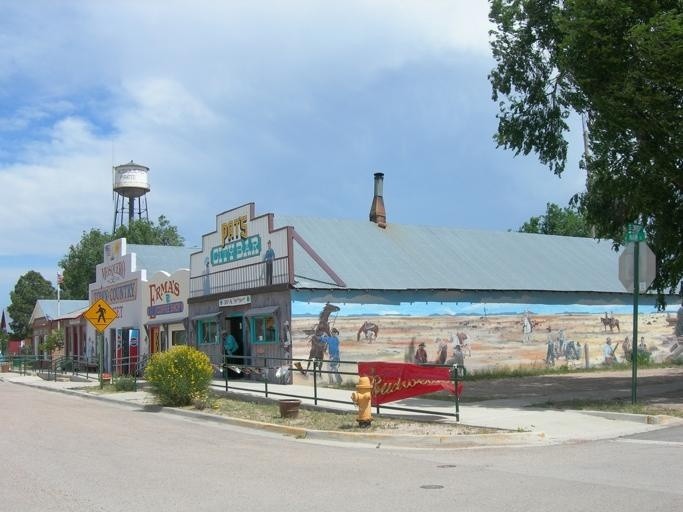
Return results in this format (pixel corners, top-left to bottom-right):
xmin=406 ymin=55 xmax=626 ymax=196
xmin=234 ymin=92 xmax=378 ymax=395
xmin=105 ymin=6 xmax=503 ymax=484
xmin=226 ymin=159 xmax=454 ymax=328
xmin=280 ymin=321 xmax=290 ymax=363
xmin=450 ymin=344 xmax=463 ymax=377
xmin=637 ymin=335 xmax=646 ymax=353
xmin=416 ymin=342 xmax=427 ymax=363
xmin=544 ymin=325 xmax=556 ymax=366
xmin=262 ymin=240 xmax=275 ymax=285
xmin=604 ymin=336 xmax=619 ymax=364
xmin=221 ymin=330 xmax=240 ymax=379
xmin=434 ymin=341 xmax=448 ymax=364
xmin=555 ymin=329 xmax=583 ymax=363
xmin=621 ymin=336 xmax=631 ymax=360
xmin=322 ymin=327 xmax=342 ymax=385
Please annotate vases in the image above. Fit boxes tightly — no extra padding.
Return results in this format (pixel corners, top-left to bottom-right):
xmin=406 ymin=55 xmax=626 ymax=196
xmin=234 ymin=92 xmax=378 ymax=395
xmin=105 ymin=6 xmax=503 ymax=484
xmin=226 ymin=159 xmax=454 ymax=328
xmin=277 ymin=398 xmax=301 ymax=419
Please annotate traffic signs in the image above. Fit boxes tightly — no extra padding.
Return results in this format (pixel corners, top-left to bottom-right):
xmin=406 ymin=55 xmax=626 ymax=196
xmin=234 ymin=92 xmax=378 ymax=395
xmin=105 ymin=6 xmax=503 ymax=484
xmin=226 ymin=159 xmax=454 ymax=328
xmin=625 ymin=232 xmax=646 ymax=243
xmin=627 ymin=222 xmax=645 ymax=232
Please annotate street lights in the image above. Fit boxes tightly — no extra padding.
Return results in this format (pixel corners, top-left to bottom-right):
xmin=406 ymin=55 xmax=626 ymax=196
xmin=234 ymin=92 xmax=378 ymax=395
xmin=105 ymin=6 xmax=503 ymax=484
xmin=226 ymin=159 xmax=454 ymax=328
xmin=55 ymin=271 xmax=64 ymax=372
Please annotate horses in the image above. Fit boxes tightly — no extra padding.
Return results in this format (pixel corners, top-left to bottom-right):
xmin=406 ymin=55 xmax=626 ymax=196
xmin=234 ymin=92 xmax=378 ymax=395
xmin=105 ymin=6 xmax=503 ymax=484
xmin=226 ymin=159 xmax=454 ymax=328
xmin=601 ymin=318 xmax=609 ymax=332
xmin=357 ymin=323 xmax=378 ymax=342
xmin=607 ymin=318 xmax=620 ymax=332
xmin=304 ymin=304 xmax=340 ymax=378
xmin=447 ymin=331 xmax=471 ymax=359
xmin=522 ymin=318 xmax=533 ymax=344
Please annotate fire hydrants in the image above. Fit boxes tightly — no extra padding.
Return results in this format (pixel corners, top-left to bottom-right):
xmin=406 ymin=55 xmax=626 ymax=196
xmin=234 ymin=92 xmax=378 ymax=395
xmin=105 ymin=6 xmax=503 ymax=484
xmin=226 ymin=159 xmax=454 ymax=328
xmin=350 ymin=376 xmax=375 ymax=429
